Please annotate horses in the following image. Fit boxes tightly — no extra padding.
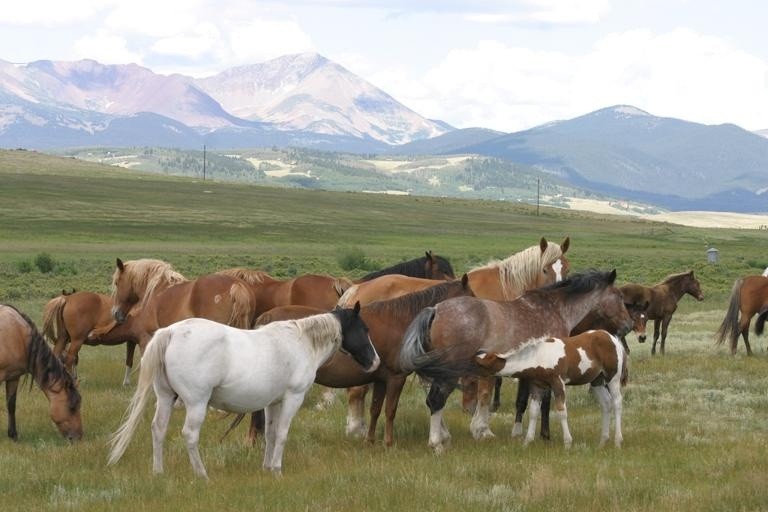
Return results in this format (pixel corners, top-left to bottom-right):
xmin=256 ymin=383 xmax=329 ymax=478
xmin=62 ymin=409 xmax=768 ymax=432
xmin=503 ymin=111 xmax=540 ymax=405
xmin=41 ymin=238 xmax=705 ymax=483
xmin=0 ymin=304 xmax=83 ymax=445
xmin=712 ymin=275 xmax=768 ymax=356
